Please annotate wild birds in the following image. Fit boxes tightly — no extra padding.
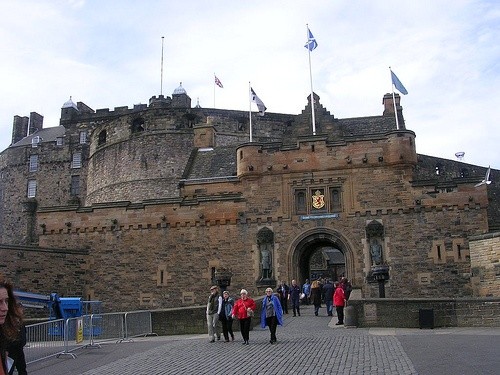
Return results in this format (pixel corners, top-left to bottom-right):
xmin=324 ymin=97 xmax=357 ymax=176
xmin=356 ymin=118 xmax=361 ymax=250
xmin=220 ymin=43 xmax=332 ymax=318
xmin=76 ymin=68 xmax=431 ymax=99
xmin=473 ymin=165 xmax=492 ymax=188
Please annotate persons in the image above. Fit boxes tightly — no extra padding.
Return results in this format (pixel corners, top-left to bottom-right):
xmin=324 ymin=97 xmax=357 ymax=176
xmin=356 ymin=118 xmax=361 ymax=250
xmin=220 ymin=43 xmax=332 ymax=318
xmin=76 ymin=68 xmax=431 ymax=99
xmin=287 ymin=280 xmax=302 ymax=317
xmin=206 ymin=285 xmax=222 ymax=343
xmin=232 ymin=289 xmax=256 ymax=345
xmin=322 ymin=279 xmax=334 ymax=316
xmin=277 ymin=280 xmax=289 ymax=314
xmin=332 ymin=283 xmax=348 ymax=325
xmin=218 ymin=290 xmax=235 ymax=343
xmin=0 ymin=278 xmax=16 ymax=375
xmin=260 ymin=288 xmax=284 ymax=344
xmin=301 ymin=275 xmax=351 ymax=308
xmin=309 ymin=280 xmax=323 ymax=316
xmin=8 ymin=299 xmax=29 ymax=375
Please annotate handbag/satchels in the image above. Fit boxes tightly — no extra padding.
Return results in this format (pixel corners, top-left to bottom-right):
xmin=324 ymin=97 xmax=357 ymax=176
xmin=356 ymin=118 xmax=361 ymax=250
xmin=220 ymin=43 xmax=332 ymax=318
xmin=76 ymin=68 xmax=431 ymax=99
xmin=247 ymin=309 xmax=254 ymax=317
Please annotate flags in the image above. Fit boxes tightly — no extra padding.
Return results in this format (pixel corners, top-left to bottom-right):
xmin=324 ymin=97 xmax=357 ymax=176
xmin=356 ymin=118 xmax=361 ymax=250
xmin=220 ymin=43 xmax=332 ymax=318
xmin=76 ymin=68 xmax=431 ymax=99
xmin=214 ymin=76 xmax=223 ymax=88
xmin=304 ymin=27 xmax=318 ymax=52
xmin=249 ymin=85 xmax=267 ymax=116
xmin=390 ymin=71 xmax=408 ymax=95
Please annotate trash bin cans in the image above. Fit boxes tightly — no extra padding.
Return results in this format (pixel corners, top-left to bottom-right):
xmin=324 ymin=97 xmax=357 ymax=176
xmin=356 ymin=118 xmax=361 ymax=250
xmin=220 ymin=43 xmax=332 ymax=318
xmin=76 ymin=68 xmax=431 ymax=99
xmin=419 ymin=308 xmax=433 ymax=329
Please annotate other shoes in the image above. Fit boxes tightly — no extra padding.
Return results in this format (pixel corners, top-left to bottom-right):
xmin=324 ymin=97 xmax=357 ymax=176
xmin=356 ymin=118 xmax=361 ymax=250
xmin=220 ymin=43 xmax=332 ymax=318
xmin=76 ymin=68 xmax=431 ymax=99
xmin=210 ymin=340 xmax=215 ymax=342
xmin=298 ymin=312 xmax=301 ymax=316
xmin=232 ymin=336 xmax=234 ymax=340
xmin=315 ymin=311 xmax=318 ymax=316
xmin=246 ymin=341 xmax=249 ymax=345
xmin=217 ymin=336 xmax=220 ymax=340
xmin=336 ymin=322 xmax=344 ymax=325
xmin=224 ymin=339 xmax=229 ymax=343
xmin=243 ymin=341 xmax=246 ymax=344
xmin=293 ymin=315 xmax=296 ymax=317
xmin=328 ymin=312 xmax=332 ymax=316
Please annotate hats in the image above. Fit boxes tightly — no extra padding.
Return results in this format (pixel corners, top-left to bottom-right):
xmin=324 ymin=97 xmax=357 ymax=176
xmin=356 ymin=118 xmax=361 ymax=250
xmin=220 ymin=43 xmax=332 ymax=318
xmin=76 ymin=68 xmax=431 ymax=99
xmin=211 ymin=285 xmax=217 ymax=290
